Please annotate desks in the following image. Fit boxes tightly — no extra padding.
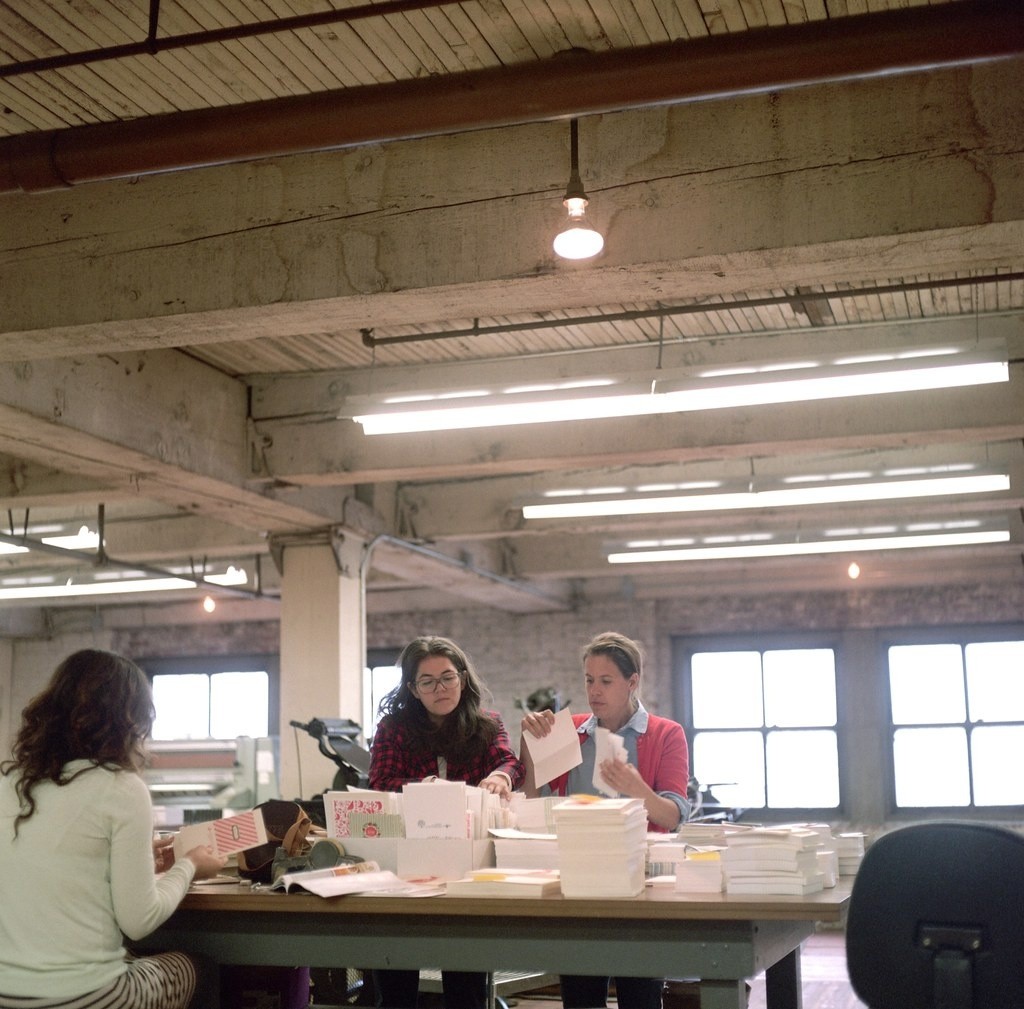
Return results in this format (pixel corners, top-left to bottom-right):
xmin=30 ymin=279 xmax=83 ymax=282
xmin=130 ymin=876 xmax=854 ymax=1009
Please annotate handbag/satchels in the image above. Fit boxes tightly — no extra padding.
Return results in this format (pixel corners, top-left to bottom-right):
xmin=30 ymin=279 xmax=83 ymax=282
xmin=237 ymin=799 xmax=327 ymax=882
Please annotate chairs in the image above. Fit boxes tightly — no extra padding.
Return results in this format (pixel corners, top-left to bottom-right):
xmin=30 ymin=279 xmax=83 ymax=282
xmin=847 ymin=822 xmax=1024 ymax=1009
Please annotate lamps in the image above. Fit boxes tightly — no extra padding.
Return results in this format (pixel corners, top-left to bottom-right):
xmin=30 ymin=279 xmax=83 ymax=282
xmin=336 ymin=267 xmax=1010 ymax=437
xmin=0 ymin=504 xmax=249 ymax=600
xmin=511 ymin=439 xmax=1011 ymax=520
xmin=548 ymin=118 xmax=605 ymax=261
xmin=606 ymin=495 xmax=1010 ymax=565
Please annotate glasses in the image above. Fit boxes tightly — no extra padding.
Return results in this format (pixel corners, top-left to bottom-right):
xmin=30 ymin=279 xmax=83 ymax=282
xmin=410 ymin=673 xmax=463 ymax=693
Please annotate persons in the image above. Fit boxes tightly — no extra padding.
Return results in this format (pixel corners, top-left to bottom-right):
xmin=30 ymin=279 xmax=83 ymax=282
xmin=357 ymin=635 xmax=525 ymax=1009
xmin=517 ymin=631 xmax=688 ymax=1009
xmin=1 ymin=650 xmax=230 ymax=1009
